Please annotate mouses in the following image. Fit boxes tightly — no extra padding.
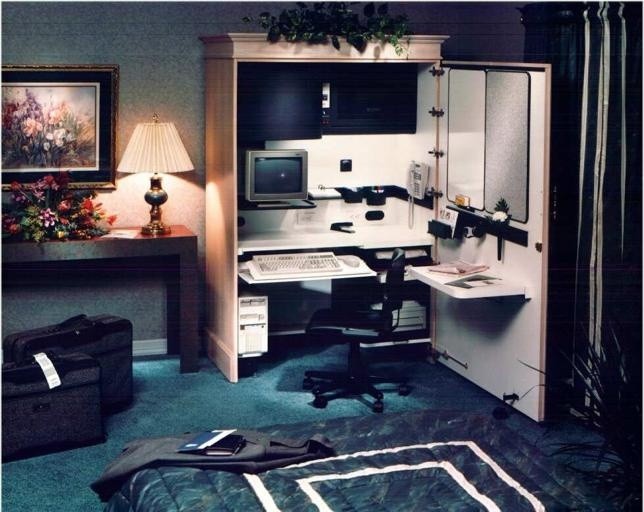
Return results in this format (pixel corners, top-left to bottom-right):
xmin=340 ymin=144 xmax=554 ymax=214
xmin=342 ymin=255 xmax=361 ymax=268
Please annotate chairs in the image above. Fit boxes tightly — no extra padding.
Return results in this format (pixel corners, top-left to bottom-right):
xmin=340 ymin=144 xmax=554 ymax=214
xmin=306 ymin=254 xmax=419 ymax=414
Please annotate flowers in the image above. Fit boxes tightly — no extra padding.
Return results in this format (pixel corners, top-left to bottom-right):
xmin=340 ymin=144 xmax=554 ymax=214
xmin=2 ymin=172 xmax=115 ymax=249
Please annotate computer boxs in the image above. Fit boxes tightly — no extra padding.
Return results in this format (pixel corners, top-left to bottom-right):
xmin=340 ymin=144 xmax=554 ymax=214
xmin=238 ymin=289 xmax=269 ymax=359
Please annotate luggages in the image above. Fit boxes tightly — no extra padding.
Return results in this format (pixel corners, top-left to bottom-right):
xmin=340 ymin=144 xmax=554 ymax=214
xmin=1 ymin=349 xmax=103 ymax=464
xmin=1 ymin=312 xmax=134 ymax=417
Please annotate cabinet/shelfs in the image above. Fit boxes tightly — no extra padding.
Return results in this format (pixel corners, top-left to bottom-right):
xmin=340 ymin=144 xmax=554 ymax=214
xmin=198 ymin=28 xmax=449 ymax=380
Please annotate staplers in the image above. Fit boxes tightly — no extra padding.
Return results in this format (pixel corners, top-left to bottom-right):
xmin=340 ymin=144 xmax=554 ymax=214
xmin=330 ymin=222 xmax=356 ymax=233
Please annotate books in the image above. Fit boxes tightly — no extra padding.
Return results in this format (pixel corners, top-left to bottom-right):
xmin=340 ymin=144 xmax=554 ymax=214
xmin=431 ymin=208 xmax=459 ymax=239
xmin=178 ymin=427 xmax=238 ymax=451
xmin=455 ymin=193 xmax=470 ymax=206
xmin=204 ymin=435 xmax=245 ymax=455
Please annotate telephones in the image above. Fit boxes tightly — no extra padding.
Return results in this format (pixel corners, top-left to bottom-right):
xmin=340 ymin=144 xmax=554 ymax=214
xmin=407 ymin=160 xmax=428 ymax=200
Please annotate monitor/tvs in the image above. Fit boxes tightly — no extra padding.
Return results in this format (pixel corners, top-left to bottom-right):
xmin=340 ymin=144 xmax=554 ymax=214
xmin=243 ymin=148 xmax=309 ymax=207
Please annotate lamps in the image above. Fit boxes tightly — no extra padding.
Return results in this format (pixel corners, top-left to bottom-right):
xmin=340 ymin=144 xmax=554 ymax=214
xmin=117 ymin=118 xmax=197 ymax=236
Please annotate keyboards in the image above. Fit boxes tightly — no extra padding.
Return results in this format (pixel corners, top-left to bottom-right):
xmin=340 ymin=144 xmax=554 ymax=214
xmin=252 ymin=251 xmax=343 ymax=276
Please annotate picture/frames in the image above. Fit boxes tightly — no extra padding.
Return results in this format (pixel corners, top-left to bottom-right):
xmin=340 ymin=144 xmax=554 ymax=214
xmin=1 ymin=65 xmax=115 ymax=187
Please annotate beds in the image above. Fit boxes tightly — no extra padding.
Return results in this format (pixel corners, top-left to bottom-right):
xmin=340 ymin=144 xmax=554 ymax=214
xmin=106 ymin=407 xmax=591 ymax=510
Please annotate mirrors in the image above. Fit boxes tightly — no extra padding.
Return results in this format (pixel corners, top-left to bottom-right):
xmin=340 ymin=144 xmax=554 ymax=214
xmin=447 ymin=67 xmax=532 ymax=221
